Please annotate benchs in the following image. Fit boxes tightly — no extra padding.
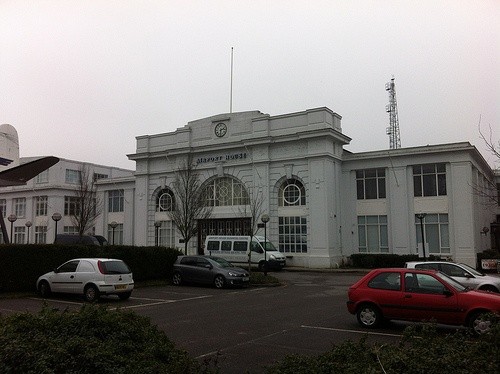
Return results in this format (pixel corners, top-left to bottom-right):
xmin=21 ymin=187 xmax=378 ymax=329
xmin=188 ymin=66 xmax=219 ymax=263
xmin=372 ymin=281 xmax=389 ymax=288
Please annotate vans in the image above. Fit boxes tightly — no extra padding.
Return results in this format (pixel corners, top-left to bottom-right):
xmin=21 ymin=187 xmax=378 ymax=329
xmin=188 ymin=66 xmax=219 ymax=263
xmin=204 ymin=235 xmax=286 ymax=273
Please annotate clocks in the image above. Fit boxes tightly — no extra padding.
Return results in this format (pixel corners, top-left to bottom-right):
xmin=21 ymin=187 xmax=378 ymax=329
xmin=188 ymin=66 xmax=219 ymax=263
xmin=214 ymin=122 xmax=227 ymax=138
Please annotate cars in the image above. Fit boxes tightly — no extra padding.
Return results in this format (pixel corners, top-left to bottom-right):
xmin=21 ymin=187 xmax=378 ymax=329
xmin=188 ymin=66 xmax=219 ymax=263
xmin=169 ymin=255 xmax=250 ymax=291
xmin=397 ymin=261 xmax=500 ymax=296
xmin=348 ymin=268 xmax=500 ymax=341
xmin=37 ymin=259 xmax=134 ymax=305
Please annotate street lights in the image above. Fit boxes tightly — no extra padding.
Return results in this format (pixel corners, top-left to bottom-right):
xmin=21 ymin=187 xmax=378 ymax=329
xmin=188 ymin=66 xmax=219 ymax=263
xmin=111 ymin=221 xmax=118 ymax=245
xmin=417 ymin=210 xmax=427 ymax=260
xmin=25 ymin=221 xmax=32 ymax=245
xmin=261 ymin=214 xmax=270 ymax=276
xmin=52 ymin=212 xmax=62 ymax=243
xmin=7 ymin=214 xmax=17 ymax=244
xmin=154 ymin=220 xmax=162 ymax=247
